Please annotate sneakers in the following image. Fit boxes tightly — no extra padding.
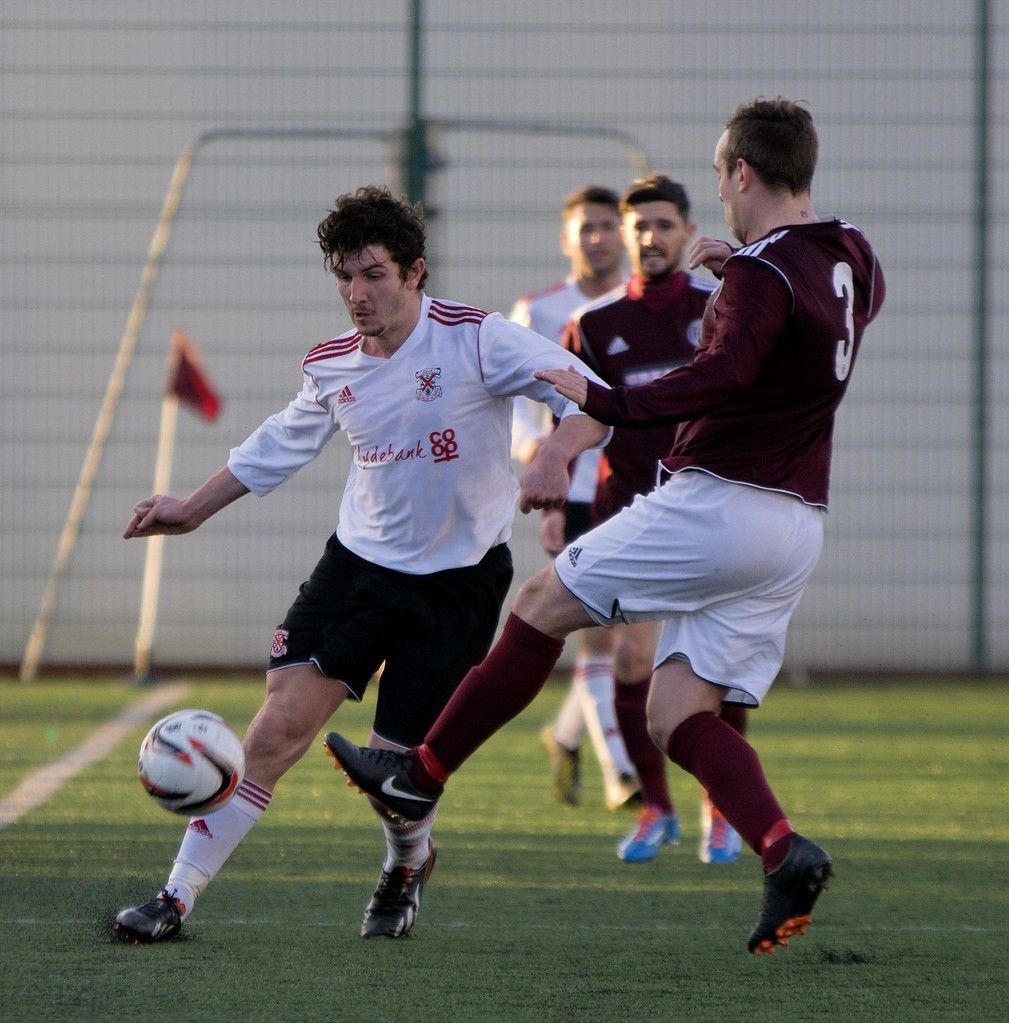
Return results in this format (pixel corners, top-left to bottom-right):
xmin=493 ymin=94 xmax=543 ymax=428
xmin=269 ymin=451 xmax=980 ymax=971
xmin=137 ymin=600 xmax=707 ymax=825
xmin=360 ymin=835 xmax=438 ymax=938
xmin=699 ymin=793 xmax=743 ymax=864
xmin=113 ymin=887 xmax=183 ymax=946
xmin=322 ymin=732 xmax=445 ymax=826
xmin=746 ymin=835 xmax=835 ymax=957
xmin=617 ymin=806 xmax=680 ymax=862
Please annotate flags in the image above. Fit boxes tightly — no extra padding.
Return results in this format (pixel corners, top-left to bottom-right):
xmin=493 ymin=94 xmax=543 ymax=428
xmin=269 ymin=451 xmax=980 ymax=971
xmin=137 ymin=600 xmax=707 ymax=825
xmin=166 ymin=351 xmax=219 ymax=423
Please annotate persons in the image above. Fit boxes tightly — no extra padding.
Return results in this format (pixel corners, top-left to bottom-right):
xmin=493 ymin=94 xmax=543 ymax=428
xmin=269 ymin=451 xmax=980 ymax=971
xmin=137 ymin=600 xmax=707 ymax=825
xmin=565 ymin=173 xmax=741 ymax=865
xmin=322 ymin=94 xmax=885 ymax=957
xmin=510 ymin=185 xmax=643 ymax=811
xmin=113 ymin=186 xmax=614 ymax=946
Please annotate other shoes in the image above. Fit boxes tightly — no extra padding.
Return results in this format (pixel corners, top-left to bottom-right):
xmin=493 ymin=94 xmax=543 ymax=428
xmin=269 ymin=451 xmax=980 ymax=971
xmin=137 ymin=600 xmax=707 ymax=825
xmin=603 ymin=773 xmax=643 ymax=811
xmin=538 ymin=722 xmax=581 ymax=808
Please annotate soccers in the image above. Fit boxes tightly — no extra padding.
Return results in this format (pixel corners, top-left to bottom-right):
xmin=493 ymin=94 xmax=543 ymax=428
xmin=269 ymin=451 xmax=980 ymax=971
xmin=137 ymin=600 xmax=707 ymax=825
xmin=137 ymin=708 xmax=246 ymax=817
